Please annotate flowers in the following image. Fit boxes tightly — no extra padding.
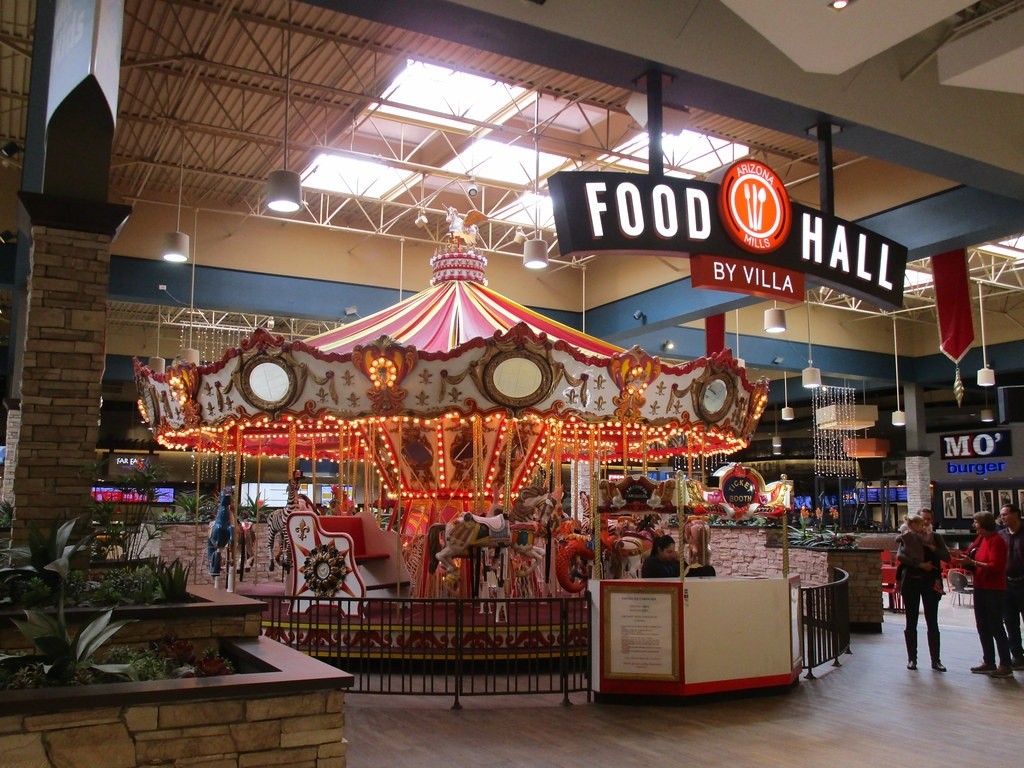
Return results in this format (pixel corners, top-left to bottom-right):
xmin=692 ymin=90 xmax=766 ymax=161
xmin=772 ymin=505 xmax=863 ymax=549
xmin=154 ymin=625 xmax=236 ymax=679
xmin=171 ymin=489 xmax=274 ymax=524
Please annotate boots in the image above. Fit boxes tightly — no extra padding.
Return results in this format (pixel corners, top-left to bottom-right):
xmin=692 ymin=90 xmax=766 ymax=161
xmin=927 ymin=631 xmax=946 ymax=671
xmin=904 ymin=630 xmax=917 ymax=669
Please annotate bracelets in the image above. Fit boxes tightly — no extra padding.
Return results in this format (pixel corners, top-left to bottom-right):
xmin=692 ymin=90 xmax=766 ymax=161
xmin=975 ymin=561 xmax=976 ymax=566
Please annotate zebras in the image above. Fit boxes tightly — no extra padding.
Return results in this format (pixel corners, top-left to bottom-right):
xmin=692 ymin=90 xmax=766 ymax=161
xmin=266 ymin=469 xmax=303 ymax=571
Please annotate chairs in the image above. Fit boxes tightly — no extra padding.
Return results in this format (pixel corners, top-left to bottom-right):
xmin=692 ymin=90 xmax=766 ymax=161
xmin=880 ymin=548 xmax=974 ymax=614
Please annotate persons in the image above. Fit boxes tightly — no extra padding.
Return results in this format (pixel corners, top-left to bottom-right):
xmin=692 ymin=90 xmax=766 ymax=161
xmin=897 ymin=507 xmax=951 ymax=671
xmin=951 ymin=511 xmax=1013 ymax=677
xmin=998 ymin=504 xmax=1024 ymax=669
xmin=892 ymin=514 xmax=947 ymax=595
xmin=641 ymin=535 xmax=680 ymax=578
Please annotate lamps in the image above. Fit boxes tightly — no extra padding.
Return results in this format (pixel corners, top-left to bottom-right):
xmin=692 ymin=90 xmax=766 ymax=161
xmin=982 ymin=386 xmax=995 ymax=422
xmin=662 ymin=339 xmax=674 ymax=349
xmin=0 ymin=303 xmax=12 ymax=314
xmin=764 ymin=300 xmax=786 ymax=333
xmin=414 ymin=210 xmax=428 ymax=229
xmin=813 ymin=378 xmax=878 ymax=430
xmin=892 ymin=319 xmax=905 ymax=426
xmin=843 ymin=381 xmax=889 ymax=458
xmin=633 ymin=309 xmax=647 ymax=320
xmin=801 ymin=290 xmax=821 ymax=388
xmin=523 ymin=92 xmax=548 ymax=269
xmin=772 ymin=404 xmax=782 ymax=455
xmin=159 ymin=126 xmax=191 ymax=263
xmin=781 ymin=371 xmax=794 ymax=420
xmin=773 ymin=356 xmax=783 ymax=363
xmin=265 ymin=0 xmax=301 ymax=212
xmin=976 ymin=281 xmax=995 ymax=386
xmin=467 ymin=179 xmax=478 ymax=198
xmin=514 ymin=226 xmax=523 ymax=244
xmin=0 ymin=229 xmax=18 ymax=244
xmin=3 ymin=141 xmax=25 ymax=156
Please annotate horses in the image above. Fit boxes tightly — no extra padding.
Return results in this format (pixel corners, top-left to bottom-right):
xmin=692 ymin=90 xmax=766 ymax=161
xmin=236 ymin=520 xmax=256 ymax=573
xmin=207 ymin=487 xmax=234 ymax=561
xmin=682 ymin=514 xmax=712 ymax=565
xmin=611 ymin=513 xmax=665 ymax=578
xmin=428 ymin=486 xmax=554 ymax=575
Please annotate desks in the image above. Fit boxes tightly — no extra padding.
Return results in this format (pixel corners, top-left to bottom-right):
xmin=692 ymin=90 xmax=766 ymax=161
xmin=881 ymin=567 xmax=906 ymax=613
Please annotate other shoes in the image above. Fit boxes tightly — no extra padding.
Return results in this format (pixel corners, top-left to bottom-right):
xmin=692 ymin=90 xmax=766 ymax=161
xmin=1011 ymin=657 xmax=1024 ymax=670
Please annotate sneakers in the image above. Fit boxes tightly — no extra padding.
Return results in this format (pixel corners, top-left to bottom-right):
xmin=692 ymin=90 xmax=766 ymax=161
xmin=991 ymin=665 xmax=1013 ymax=677
xmin=970 ymin=662 xmax=997 ymax=674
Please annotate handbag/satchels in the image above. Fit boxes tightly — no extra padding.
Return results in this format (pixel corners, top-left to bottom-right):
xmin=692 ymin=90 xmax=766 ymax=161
xmin=962 ymin=547 xmax=977 ymax=572
